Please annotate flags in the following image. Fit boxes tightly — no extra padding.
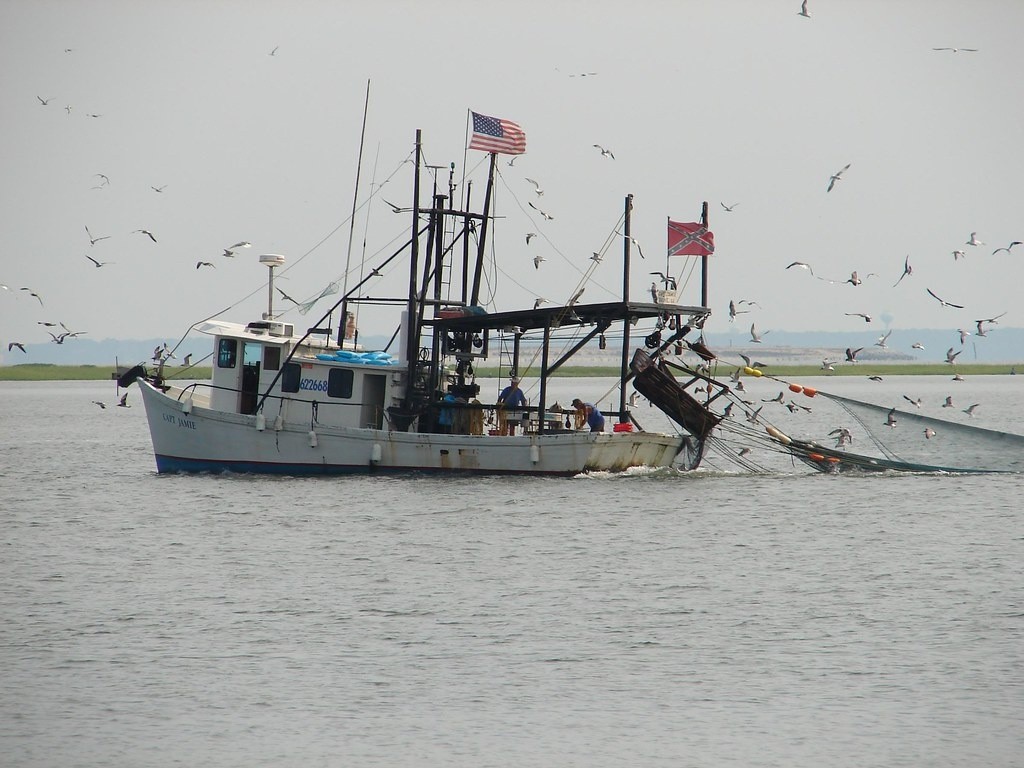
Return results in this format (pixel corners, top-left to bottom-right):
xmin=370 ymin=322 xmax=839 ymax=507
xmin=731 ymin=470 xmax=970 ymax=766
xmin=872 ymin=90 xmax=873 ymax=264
xmin=668 ymin=219 xmax=715 ymax=256
xmin=468 ymin=110 xmax=525 ymax=154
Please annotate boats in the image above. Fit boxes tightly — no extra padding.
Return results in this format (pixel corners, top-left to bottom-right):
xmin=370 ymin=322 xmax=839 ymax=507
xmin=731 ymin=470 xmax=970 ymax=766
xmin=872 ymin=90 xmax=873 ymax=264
xmin=117 ymin=76 xmax=731 ymax=478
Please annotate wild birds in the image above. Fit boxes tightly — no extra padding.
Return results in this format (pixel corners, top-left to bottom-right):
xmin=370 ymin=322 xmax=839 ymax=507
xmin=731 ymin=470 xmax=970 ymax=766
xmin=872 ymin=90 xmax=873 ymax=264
xmin=0 ymin=0 xmax=1023 ymax=448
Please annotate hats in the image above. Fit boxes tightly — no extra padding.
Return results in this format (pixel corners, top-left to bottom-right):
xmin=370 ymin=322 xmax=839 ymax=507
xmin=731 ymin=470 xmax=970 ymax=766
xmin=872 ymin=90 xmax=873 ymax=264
xmin=509 ymin=377 xmax=520 ymax=383
xmin=571 ymin=399 xmax=582 ymax=406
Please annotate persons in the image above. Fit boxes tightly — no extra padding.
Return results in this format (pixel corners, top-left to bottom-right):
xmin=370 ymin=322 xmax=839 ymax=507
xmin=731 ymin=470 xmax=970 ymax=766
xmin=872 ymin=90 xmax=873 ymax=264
xmin=497 ymin=376 xmax=527 ymax=436
xmin=571 ymin=399 xmax=605 ymax=432
